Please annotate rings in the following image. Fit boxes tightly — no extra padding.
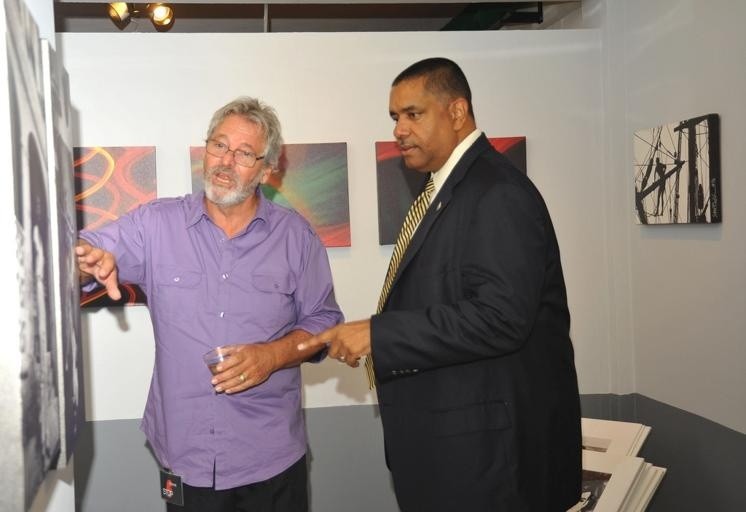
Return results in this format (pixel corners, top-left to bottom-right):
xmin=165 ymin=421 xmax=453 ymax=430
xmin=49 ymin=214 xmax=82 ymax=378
xmin=238 ymin=374 xmax=244 ymax=382
xmin=336 ymin=353 xmax=345 ymax=361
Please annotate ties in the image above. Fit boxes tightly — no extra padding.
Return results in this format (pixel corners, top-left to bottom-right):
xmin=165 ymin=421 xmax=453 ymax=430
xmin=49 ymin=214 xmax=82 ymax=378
xmin=364 ymin=176 xmax=435 ymax=391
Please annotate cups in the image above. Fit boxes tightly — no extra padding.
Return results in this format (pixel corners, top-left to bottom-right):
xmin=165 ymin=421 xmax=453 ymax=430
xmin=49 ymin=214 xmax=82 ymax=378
xmin=204 ymin=344 xmax=238 ymax=375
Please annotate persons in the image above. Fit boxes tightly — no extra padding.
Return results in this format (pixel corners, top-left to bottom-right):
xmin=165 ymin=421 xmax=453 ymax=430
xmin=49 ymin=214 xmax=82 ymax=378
xmin=294 ymin=55 xmax=587 ymax=512
xmin=652 ymin=157 xmax=668 ymax=218
xmin=73 ymin=93 xmax=346 ymax=512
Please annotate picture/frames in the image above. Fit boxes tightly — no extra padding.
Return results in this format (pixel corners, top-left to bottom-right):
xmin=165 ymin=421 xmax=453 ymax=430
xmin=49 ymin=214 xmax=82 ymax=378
xmin=567 ymin=417 xmax=668 ymax=512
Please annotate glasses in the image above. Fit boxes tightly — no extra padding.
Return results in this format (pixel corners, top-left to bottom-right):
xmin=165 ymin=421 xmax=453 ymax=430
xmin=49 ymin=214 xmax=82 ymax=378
xmin=205 ymin=136 xmax=264 ymax=169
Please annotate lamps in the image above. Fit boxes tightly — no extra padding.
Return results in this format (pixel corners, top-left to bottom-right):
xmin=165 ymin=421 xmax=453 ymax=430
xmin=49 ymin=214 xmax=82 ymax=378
xmin=108 ymin=1 xmax=175 ymax=28
